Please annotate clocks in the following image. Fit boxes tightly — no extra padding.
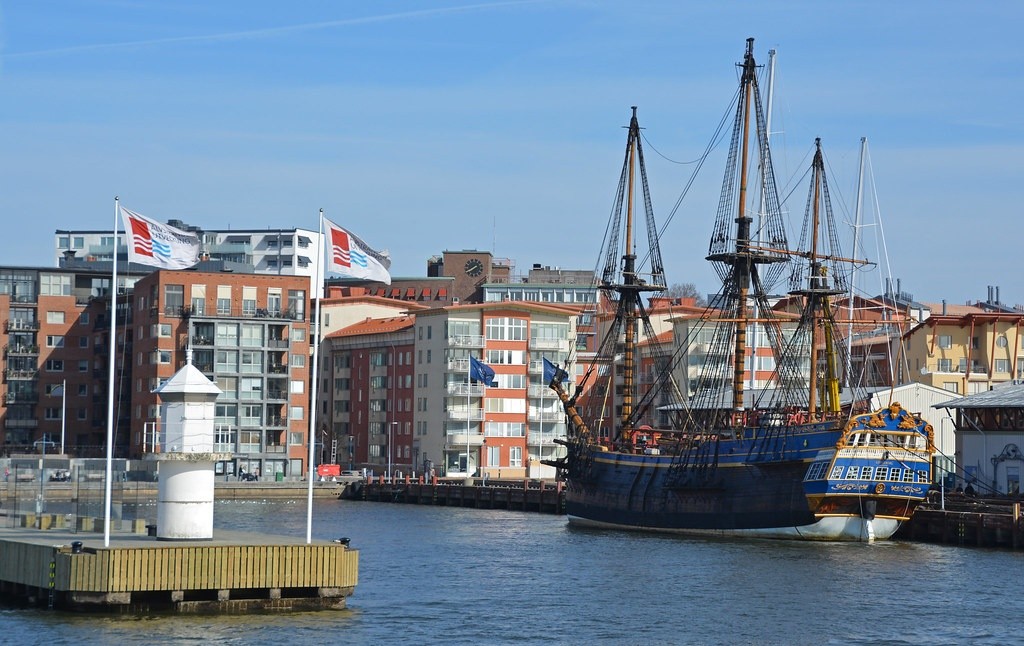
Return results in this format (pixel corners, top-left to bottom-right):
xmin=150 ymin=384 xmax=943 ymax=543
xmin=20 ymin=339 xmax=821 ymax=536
xmin=465 ymin=259 xmax=483 ymax=278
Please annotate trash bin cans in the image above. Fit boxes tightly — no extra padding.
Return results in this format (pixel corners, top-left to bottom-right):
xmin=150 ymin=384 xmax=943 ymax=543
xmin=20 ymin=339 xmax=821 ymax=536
xmin=275 ymin=472 xmax=283 ymax=481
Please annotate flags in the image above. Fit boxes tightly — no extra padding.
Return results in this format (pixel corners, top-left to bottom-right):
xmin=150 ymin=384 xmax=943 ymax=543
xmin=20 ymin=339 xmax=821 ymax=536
xmin=542 ymin=356 xmax=572 ymax=384
xmin=469 ymin=354 xmax=496 ymax=387
xmin=322 ymin=214 xmax=393 ymax=287
xmin=117 ymin=204 xmax=200 ymax=271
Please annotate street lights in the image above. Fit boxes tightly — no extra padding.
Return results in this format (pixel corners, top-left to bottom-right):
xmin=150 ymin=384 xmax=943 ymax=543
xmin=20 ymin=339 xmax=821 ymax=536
xmin=48 ymin=378 xmax=66 ymax=454
xmin=348 ymin=434 xmax=355 ymax=470
xmin=386 ymin=421 xmax=398 ymax=484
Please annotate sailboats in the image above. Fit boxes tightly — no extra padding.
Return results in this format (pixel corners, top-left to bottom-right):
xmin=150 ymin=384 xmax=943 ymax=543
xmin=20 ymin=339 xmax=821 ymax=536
xmin=537 ymin=34 xmax=939 ymax=547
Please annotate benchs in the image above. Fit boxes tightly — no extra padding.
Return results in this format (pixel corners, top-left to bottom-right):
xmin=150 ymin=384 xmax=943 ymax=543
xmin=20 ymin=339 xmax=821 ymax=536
xmin=50 ymin=473 xmax=72 ymax=482
xmin=16 ymin=474 xmax=35 ymax=482
xmin=87 ymin=473 xmax=106 ymax=481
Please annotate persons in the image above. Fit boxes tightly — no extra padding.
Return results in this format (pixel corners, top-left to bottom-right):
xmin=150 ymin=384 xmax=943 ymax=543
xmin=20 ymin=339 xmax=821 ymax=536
xmin=57 ymin=469 xmax=61 ymax=476
xmin=424 ymin=458 xmax=432 ymax=484
xmin=238 ymin=464 xmax=243 ymax=481
xmin=956 ymin=482 xmax=975 ymax=497
xmin=255 ymin=467 xmax=259 ymax=481
